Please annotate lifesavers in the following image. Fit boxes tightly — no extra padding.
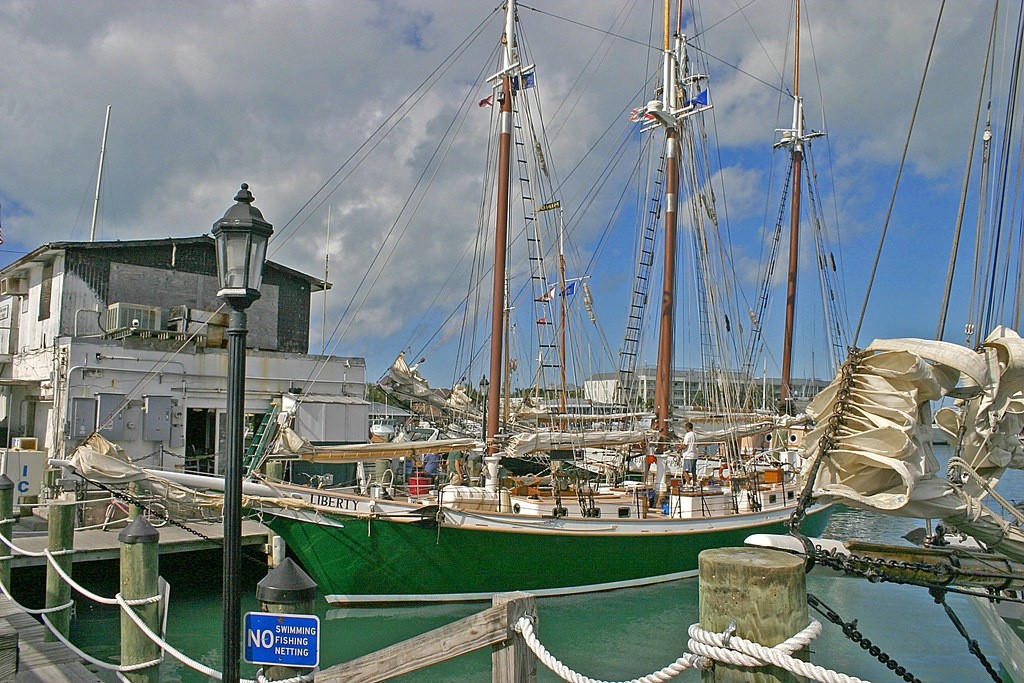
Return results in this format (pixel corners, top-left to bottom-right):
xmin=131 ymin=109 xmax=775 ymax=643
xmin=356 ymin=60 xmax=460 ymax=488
xmin=719 ymin=464 xmax=732 ymax=480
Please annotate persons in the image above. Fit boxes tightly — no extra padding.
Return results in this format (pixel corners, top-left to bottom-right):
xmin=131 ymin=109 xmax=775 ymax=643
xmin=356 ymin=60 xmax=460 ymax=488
xmin=446 ymin=452 xmax=464 ymax=486
xmin=421 ymin=452 xmax=441 ymax=485
xmin=677 ymin=422 xmax=699 ymax=490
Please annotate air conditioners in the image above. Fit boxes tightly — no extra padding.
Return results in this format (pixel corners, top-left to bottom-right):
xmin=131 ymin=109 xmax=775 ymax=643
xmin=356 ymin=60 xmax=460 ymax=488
xmin=107 ymin=302 xmax=162 ymax=331
xmin=1 ymin=276 xmax=29 ymax=295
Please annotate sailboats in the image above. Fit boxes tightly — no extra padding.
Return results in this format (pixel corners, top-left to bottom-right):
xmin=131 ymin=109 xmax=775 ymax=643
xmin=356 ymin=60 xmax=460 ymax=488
xmin=744 ymin=0 xmax=1024 ymax=683
xmin=45 ymin=0 xmax=835 ymax=608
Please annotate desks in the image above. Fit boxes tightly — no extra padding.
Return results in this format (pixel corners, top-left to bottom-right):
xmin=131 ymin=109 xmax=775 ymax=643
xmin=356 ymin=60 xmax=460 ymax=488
xmin=739 ymin=482 xmax=797 ymax=513
xmin=669 ymin=489 xmax=726 ymax=519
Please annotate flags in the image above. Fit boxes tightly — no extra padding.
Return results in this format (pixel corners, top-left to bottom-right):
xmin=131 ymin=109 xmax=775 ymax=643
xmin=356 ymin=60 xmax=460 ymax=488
xmin=538 ymin=287 xmax=555 ymax=300
xmin=684 ymin=87 xmax=709 ymax=105
xmin=629 ymin=107 xmax=655 ymax=121
xmin=478 ymin=95 xmax=493 ymax=110
xmin=559 ymin=282 xmax=575 ymax=296
xmin=513 ymin=72 xmax=535 ymax=90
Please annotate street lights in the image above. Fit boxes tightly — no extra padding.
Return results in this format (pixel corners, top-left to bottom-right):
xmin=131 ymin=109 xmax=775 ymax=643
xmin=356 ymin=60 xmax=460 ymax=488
xmin=211 ymin=184 xmax=274 ymax=683
